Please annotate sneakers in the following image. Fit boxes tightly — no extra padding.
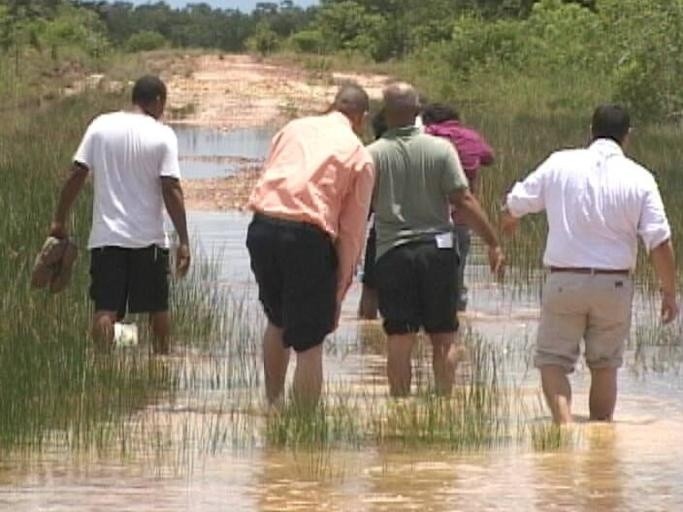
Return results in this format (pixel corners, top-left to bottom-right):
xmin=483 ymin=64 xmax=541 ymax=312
xmin=50 ymin=242 xmax=78 ymax=292
xmin=29 ymin=238 xmax=65 ymax=288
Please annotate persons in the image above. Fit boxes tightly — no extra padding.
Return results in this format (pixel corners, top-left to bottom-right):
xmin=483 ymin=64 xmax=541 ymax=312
xmin=241 ymin=82 xmax=378 ymax=418
xmin=502 ymin=103 xmax=678 ymax=426
xmin=364 ymin=80 xmax=507 ymax=400
xmin=422 ymin=100 xmax=497 ymax=314
xmin=356 ymin=107 xmax=390 ymax=320
xmin=48 ymin=73 xmax=191 ymax=356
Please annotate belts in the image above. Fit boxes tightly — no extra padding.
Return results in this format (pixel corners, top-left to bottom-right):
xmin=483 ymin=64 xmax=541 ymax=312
xmin=550 ymin=266 xmax=631 ymax=274
